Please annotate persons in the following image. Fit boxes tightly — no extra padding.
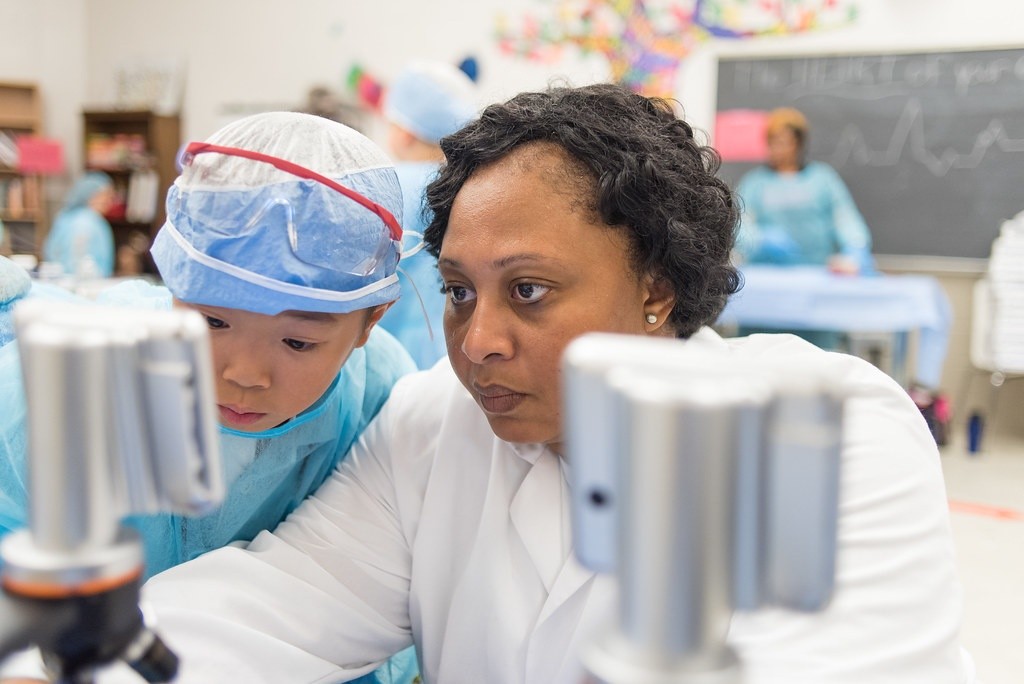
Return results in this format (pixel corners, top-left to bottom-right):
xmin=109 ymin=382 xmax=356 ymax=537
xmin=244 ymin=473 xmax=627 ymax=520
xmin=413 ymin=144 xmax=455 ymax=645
xmin=732 ymin=109 xmax=875 ymax=353
xmin=0 ymin=73 xmax=466 ymax=684
xmin=0 ymin=80 xmax=962 ymax=684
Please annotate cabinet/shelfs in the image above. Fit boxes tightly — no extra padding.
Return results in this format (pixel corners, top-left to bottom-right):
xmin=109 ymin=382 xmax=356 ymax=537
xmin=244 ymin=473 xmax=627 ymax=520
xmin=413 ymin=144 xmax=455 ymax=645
xmin=81 ymin=106 xmax=183 ymax=284
xmin=1 ymin=79 xmax=49 ymax=279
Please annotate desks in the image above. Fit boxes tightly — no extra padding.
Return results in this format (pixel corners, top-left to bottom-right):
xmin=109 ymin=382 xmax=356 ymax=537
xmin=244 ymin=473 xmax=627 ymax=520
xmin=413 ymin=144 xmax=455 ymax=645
xmin=714 ymin=266 xmax=950 ymax=393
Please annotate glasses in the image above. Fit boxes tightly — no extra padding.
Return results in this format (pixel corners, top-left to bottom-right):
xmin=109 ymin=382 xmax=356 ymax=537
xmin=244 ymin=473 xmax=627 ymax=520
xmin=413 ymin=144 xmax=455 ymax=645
xmin=173 ymin=142 xmax=404 ymax=276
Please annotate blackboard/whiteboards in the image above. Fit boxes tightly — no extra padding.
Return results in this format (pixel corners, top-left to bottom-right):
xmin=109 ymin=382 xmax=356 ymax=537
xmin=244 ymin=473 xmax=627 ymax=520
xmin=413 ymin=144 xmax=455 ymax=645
xmin=700 ymin=45 xmax=1024 ymax=271
